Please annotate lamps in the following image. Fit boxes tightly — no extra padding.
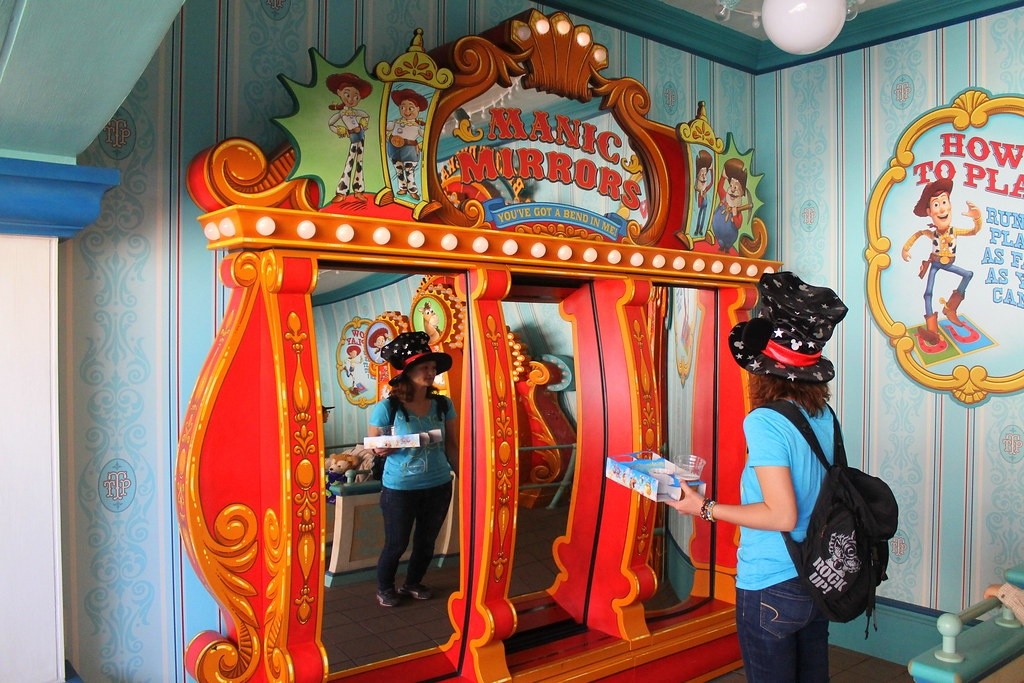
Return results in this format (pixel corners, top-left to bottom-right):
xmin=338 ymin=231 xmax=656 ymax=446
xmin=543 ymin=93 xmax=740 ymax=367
xmin=712 ymin=0 xmax=866 ymax=55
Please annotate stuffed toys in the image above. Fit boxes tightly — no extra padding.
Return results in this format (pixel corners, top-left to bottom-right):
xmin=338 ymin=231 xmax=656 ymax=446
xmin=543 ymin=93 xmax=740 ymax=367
xmin=325 ymin=452 xmax=362 ymax=505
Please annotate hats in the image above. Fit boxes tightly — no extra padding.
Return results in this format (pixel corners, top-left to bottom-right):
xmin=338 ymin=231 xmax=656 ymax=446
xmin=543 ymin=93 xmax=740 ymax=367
xmin=727 ymin=272 xmax=848 ymax=380
xmin=380 ymin=331 xmax=453 ymax=384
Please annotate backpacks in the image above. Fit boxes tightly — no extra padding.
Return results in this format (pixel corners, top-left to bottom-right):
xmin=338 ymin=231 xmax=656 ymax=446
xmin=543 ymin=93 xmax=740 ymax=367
xmin=371 ymin=395 xmax=448 ymax=481
xmin=750 ymin=399 xmax=898 ymax=638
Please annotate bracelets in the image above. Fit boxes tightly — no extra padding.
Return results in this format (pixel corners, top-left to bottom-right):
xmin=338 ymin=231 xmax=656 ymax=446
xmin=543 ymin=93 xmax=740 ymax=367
xmin=699 ymin=499 xmax=719 ymax=523
xmin=372 ymin=449 xmax=381 ymax=456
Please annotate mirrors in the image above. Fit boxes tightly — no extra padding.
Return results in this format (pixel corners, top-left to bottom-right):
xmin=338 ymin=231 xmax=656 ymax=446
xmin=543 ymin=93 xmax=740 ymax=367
xmin=308 ymin=258 xmax=466 ymax=681
xmin=640 ymin=279 xmax=719 ymax=620
xmin=502 ymin=269 xmax=610 ymax=657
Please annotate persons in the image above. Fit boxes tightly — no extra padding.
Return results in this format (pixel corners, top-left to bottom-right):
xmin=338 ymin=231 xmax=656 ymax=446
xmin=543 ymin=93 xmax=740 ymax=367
xmin=984 ymin=582 xmax=1024 ymax=627
xmin=666 ymin=271 xmax=851 ymax=683
xmin=368 ymin=333 xmax=462 ymax=608
